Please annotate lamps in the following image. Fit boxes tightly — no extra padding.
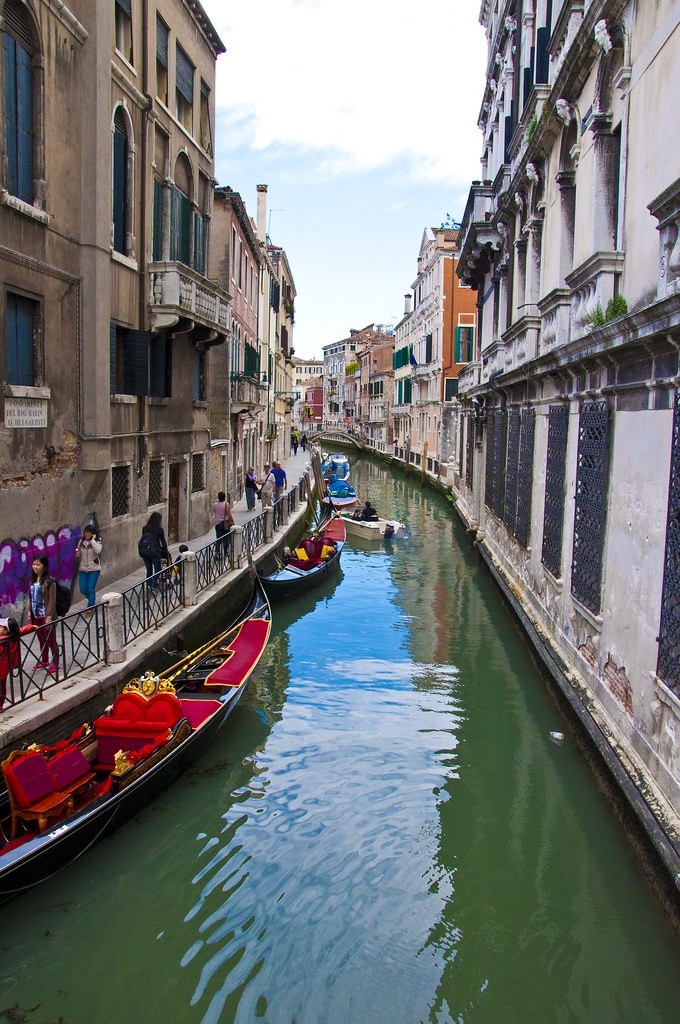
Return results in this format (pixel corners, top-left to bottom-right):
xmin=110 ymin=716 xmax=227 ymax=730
xmin=230 ymin=371 xmax=269 ymax=386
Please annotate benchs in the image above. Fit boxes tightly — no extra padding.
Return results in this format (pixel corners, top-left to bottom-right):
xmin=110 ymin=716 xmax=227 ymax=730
xmin=46 ymin=745 xmax=97 ymax=812
xmin=92 ymin=669 xmax=184 ymax=766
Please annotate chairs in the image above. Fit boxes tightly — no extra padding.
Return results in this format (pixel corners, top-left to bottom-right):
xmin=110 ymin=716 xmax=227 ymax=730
xmin=0 ymin=749 xmax=74 ymax=837
xmin=315 ymin=537 xmax=334 ymax=561
xmin=299 ymin=540 xmax=317 ymax=558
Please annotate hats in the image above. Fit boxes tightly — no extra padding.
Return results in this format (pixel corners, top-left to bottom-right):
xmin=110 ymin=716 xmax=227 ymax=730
xmin=0 ymin=617 xmax=10 ymax=631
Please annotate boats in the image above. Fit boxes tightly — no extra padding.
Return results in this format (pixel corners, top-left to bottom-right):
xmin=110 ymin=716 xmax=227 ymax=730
xmin=322 ymin=474 xmax=360 ymax=512
xmin=0 ymin=547 xmax=272 ymax=896
xmin=321 ymin=451 xmax=352 ymax=486
xmin=329 ymin=508 xmax=406 ymax=540
xmin=259 ymin=511 xmax=346 ymax=596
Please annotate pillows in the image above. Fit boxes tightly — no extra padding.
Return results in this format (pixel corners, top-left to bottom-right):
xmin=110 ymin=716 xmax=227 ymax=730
xmin=320 ymin=544 xmax=334 ymax=559
xmin=295 ymin=547 xmax=310 ymax=561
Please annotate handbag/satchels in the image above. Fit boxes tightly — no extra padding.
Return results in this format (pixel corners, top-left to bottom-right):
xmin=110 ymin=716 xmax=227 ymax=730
xmin=255 ymin=489 xmax=262 ymax=499
xmin=223 ymin=502 xmax=235 ymax=530
xmin=74 ymin=558 xmax=80 ymax=570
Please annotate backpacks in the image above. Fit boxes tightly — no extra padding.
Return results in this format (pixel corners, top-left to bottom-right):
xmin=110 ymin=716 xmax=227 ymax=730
xmin=138 ymin=526 xmax=159 ymax=557
xmin=43 ymin=579 xmax=72 ymax=617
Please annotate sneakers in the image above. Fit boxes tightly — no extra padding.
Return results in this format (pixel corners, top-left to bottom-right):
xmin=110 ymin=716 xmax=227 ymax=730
xmin=31 ymin=661 xmax=51 ymax=671
xmin=46 ymin=662 xmax=61 ymax=675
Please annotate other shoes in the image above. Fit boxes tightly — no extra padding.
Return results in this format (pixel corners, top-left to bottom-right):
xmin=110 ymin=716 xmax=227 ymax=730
xmin=253 ymin=507 xmax=256 ymax=511
xmin=151 ymin=587 xmax=157 ymax=595
xmin=86 ymin=608 xmax=95 ymax=618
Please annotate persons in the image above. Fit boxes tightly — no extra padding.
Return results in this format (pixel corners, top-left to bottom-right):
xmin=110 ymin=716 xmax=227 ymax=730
xmin=290 ymin=425 xmax=300 ymax=456
xmin=75 ymin=526 xmax=103 ymax=618
xmin=27 ymin=555 xmax=60 ymax=674
xmin=243 ymin=461 xmax=287 ymax=512
xmin=352 ymin=501 xmax=378 ymax=522
xmin=301 ymin=435 xmax=307 ymax=452
xmin=0 ymin=617 xmax=38 ymax=713
xmin=212 ymin=492 xmax=235 ymax=561
xmin=139 ymin=512 xmax=168 ymax=595
xmin=172 ymin=545 xmax=188 ymax=587
